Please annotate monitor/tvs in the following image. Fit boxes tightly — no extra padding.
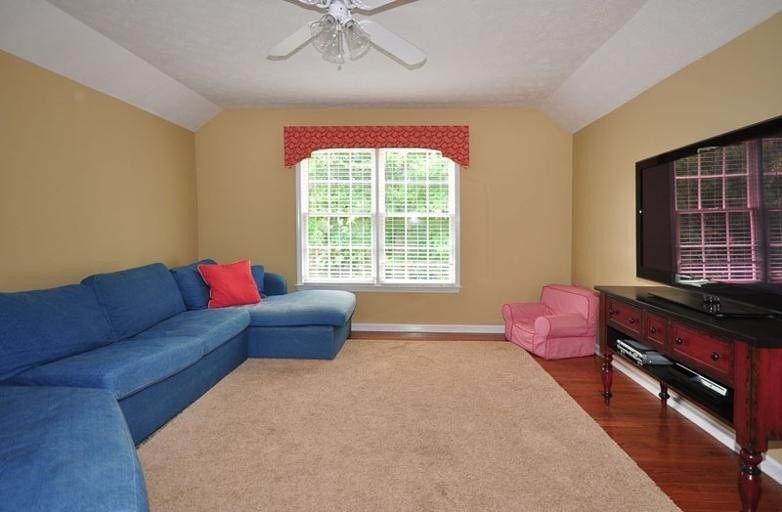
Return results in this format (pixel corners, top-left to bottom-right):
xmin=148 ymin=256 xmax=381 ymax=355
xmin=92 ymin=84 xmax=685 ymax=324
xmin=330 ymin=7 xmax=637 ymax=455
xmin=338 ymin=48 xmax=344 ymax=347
xmin=635 ymin=116 xmax=782 ymax=320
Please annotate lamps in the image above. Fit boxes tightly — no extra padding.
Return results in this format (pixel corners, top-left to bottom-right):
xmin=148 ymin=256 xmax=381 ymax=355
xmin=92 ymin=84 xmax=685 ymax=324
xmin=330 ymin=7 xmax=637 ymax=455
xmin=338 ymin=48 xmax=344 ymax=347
xmin=312 ymin=3 xmax=370 ymax=63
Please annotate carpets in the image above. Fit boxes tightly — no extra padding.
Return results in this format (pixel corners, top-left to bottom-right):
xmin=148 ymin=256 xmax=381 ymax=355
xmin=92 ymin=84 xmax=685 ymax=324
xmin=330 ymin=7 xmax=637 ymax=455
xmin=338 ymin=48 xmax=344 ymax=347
xmin=135 ymin=340 xmax=684 ymax=512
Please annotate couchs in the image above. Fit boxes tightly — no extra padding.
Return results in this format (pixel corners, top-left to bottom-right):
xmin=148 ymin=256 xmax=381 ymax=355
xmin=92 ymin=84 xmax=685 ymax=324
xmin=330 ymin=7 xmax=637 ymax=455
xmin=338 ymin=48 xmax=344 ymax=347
xmin=502 ymin=284 xmax=600 ymax=361
xmin=0 ymin=263 xmax=250 ymax=446
xmin=1 ymin=387 xmax=150 ymax=511
xmin=171 ymin=258 xmax=356 ymax=361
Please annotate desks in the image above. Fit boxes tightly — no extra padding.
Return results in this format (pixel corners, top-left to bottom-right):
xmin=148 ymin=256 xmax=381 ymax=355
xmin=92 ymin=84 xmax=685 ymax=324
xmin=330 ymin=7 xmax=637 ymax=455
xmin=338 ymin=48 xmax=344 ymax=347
xmin=594 ymin=285 xmax=778 ymax=511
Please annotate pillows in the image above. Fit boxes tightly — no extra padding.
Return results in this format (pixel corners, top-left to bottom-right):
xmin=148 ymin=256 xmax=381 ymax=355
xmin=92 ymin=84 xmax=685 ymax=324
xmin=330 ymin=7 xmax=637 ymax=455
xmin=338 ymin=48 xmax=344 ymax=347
xmin=198 ymin=258 xmax=262 ymax=309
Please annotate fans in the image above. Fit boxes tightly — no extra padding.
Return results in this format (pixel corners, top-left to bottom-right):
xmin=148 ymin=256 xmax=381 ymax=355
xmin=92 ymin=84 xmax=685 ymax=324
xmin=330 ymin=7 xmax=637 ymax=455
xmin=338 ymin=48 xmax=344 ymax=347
xmin=253 ymin=1 xmax=429 ymax=64
